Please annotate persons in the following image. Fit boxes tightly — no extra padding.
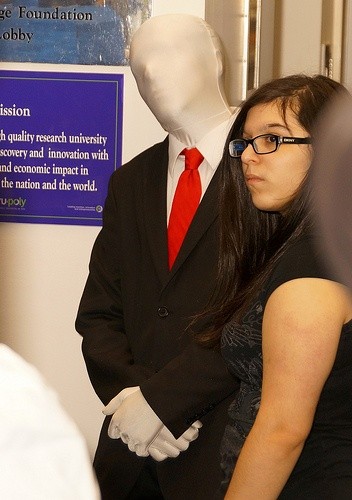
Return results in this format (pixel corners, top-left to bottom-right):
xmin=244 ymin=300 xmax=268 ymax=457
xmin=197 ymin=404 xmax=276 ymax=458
xmin=180 ymin=72 xmax=352 ymax=500
xmin=75 ymin=14 xmax=243 ymax=500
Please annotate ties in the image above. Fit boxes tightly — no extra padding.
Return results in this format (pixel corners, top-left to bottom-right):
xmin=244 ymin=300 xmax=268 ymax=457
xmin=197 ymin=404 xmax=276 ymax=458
xmin=167 ymin=147 xmax=205 ymax=273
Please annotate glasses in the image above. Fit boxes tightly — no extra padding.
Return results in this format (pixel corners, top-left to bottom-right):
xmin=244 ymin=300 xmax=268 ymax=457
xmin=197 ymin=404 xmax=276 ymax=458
xmin=229 ymin=133 xmax=315 ymax=158
